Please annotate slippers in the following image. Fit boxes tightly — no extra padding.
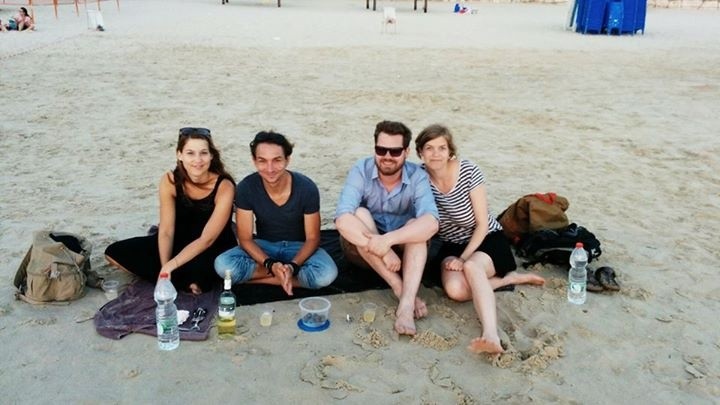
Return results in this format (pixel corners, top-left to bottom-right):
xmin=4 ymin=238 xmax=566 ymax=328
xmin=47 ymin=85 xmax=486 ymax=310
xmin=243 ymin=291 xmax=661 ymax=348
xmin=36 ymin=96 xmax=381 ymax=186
xmin=597 ymin=267 xmax=619 ymax=291
xmin=586 ymin=267 xmax=603 ymax=291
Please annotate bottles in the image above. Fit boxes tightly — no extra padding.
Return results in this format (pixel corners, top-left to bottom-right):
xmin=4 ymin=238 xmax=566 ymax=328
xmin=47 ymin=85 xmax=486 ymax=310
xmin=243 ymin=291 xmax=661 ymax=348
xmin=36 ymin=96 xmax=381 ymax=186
xmin=153 ymin=272 xmax=180 ymax=351
xmin=568 ymin=243 xmax=587 ymax=304
xmin=217 ymin=268 xmax=236 ymax=338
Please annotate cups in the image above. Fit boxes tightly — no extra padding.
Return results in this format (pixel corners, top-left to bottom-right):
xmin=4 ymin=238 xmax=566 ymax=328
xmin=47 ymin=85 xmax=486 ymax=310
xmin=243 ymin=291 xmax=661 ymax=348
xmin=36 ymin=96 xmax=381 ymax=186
xmin=363 ymin=303 xmax=377 ymax=322
xmin=103 ymin=280 xmax=119 ymax=300
xmin=259 ymin=307 xmax=273 ymax=327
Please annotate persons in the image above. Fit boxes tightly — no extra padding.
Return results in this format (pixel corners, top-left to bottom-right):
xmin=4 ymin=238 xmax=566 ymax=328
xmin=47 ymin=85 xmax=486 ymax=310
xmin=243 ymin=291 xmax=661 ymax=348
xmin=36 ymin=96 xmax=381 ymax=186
xmin=103 ymin=128 xmax=239 ymax=296
xmin=415 ymin=124 xmax=544 ymax=353
xmin=334 ymin=120 xmax=439 ymax=336
xmin=17 ymin=7 xmax=34 ymax=30
xmin=214 ymin=131 xmax=338 ymax=296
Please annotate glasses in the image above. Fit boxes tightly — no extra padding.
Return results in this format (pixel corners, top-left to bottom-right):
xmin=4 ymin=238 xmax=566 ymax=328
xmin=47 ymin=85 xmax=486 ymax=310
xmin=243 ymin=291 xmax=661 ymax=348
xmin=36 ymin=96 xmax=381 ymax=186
xmin=375 ymin=145 xmax=404 ymax=157
xmin=180 ymin=128 xmax=211 ymax=136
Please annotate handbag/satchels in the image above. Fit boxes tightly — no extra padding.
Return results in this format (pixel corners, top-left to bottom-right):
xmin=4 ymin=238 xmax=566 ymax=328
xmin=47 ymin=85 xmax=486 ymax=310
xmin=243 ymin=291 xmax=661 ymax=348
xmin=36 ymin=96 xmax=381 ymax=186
xmin=13 ymin=229 xmax=104 ymax=305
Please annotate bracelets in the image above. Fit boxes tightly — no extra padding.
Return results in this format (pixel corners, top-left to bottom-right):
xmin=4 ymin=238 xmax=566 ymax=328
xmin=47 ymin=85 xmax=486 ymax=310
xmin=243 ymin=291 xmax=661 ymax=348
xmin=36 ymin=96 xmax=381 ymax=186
xmin=264 ymin=258 xmax=277 ymax=277
xmin=288 ymin=262 xmax=299 ymax=277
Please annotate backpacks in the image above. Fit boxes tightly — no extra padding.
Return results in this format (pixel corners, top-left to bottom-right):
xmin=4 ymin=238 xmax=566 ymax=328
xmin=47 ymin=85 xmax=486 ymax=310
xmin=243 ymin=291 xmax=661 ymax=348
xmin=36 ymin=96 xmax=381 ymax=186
xmin=495 ymin=192 xmax=602 ymax=267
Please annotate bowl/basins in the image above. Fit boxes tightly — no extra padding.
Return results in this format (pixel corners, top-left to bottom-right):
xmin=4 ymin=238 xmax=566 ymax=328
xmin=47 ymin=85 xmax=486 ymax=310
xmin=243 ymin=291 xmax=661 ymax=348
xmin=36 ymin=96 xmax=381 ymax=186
xmin=299 ymin=296 xmax=331 ymax=327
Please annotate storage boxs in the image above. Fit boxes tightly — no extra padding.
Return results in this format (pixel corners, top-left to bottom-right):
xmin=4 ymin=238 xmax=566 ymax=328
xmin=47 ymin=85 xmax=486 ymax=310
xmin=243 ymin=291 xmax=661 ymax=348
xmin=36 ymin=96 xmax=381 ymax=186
xmin=570 ymin=0 xmax=647 ymax=36
